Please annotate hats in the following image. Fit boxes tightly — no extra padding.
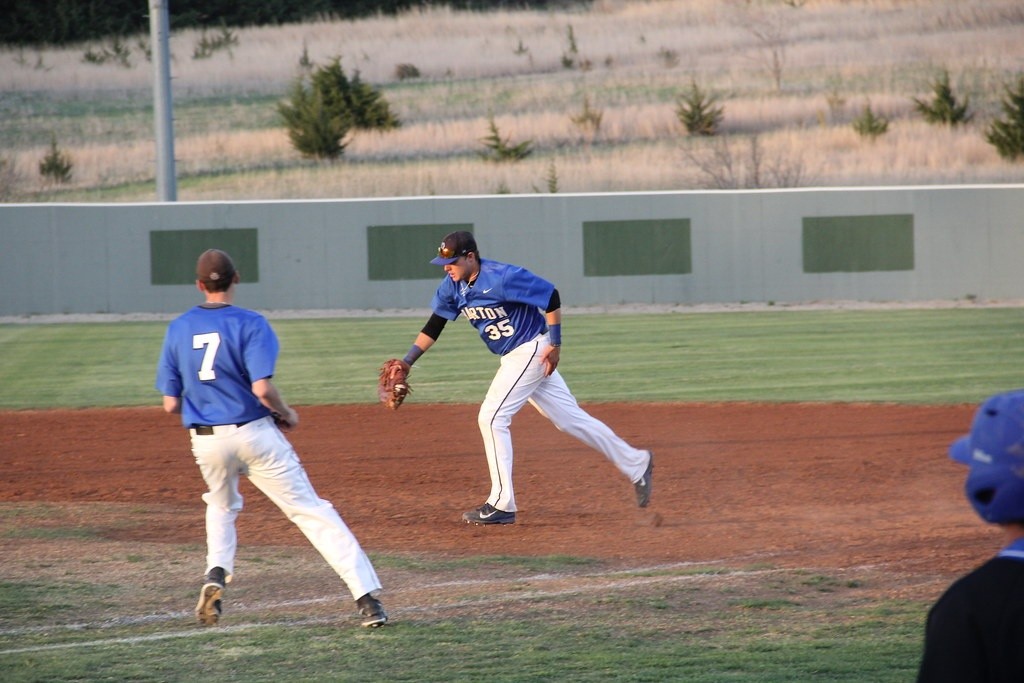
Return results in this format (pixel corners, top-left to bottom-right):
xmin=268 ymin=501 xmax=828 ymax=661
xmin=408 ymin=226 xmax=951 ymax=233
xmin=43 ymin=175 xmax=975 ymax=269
xmin=196 ymin=249 xmax=234 ymax=281
xmin=429 ymin=230 xmax=477 ymax=266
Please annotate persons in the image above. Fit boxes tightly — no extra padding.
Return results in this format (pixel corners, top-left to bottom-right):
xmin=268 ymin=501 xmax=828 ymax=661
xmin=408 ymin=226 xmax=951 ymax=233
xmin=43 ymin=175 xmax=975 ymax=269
xmin=898 ymin=388 xmax=1024 ymax=683
xmin=153 ymin=246 xmax=389 ymax=627
xmin=379 ymin=232 xmax=653 ymax=525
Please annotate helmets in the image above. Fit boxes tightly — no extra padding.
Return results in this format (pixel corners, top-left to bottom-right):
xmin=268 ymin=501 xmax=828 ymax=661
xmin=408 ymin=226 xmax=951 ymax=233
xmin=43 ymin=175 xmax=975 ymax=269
xmin=949 ymin=388 xmax=1024 ymax=525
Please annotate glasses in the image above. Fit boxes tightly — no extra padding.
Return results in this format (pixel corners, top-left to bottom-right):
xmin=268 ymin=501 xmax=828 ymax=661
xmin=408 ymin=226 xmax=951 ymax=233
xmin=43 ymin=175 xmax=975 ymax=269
xmin=436 ymin=245 xmax=457 ymax=259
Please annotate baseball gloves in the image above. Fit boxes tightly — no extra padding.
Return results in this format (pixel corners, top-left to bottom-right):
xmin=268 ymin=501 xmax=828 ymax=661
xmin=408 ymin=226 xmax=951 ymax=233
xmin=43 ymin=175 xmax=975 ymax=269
xmin=378 ymin=357 xmax=409 ymax=411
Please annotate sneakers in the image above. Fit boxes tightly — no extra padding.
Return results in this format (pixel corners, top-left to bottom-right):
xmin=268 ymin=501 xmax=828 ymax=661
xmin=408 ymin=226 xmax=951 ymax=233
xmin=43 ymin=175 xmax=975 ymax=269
xmin=194 ymin=577 xmax=225 ymax=626
xmin=634 ymin=450 xmax=651 ymax=507
xmin=462 ymin=502 xmax=515 ymax=524
xmin=358 ymin=599 xmax=387 ymax=627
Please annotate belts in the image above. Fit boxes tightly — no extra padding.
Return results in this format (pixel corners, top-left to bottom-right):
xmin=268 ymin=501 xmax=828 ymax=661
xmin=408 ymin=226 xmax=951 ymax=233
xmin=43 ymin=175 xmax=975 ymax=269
xmin=196 ymin=421 xmax=250 ymax=434
xmin=540 ymin=325 xmax=549 ymax=335
